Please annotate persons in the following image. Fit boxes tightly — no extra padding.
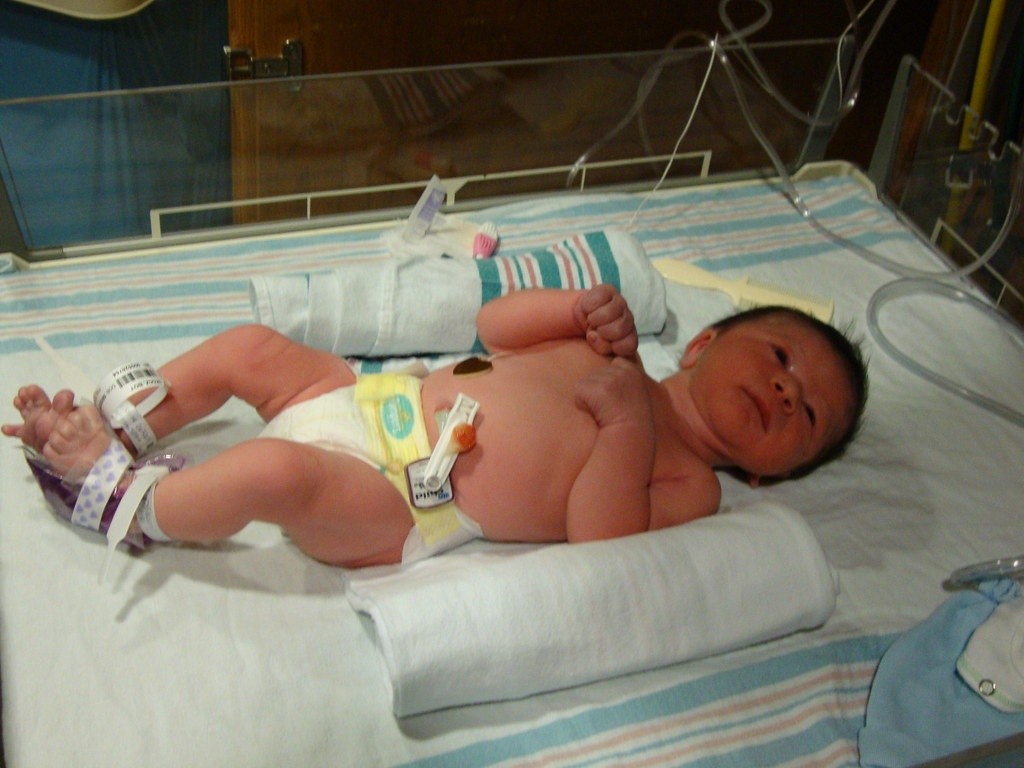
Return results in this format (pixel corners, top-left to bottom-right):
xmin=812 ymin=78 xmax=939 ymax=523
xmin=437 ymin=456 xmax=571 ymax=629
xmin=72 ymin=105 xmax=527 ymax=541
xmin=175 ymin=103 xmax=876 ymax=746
xmin=0 ymin=285 xmax=869 ymax=569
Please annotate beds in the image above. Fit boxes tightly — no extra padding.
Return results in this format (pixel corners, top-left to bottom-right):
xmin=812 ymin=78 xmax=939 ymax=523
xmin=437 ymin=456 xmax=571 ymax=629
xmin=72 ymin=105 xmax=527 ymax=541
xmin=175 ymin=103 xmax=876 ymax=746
xmin=0 ymin=149 xmax=1024 ymax=768
